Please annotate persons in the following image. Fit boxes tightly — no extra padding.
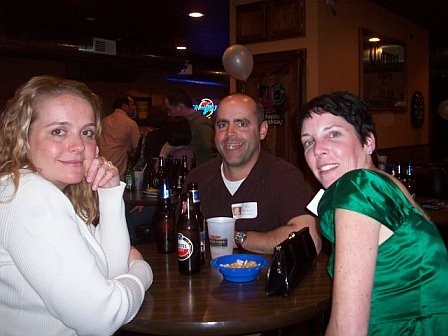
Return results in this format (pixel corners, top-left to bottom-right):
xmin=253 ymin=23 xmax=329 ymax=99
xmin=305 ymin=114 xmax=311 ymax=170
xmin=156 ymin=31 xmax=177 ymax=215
xmin=97 ymin=94 xmax=141 ymax=175
xmin=126 ymin=205 xmax=158 ymax=238
xmin=175 ymin=93 xmax=322 ymax=258
xmin=296 ymin=92 xmax=448 ymax=336
xmin=233 ymin=207 xmax=241 ymax=215
xmin=151 ymin=89 xmax=215 ymax=176
xmin=0 ymin=76 xmax=153 ymax=336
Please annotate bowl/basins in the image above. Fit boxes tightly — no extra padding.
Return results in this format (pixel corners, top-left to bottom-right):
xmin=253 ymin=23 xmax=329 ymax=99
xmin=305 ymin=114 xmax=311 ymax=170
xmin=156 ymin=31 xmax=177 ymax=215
xmin=210 ymin=254 xmax=270 ymax=282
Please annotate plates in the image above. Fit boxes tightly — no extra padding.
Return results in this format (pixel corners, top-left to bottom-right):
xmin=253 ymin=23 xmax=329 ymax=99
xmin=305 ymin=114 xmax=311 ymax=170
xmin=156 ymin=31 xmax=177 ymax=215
xmin=142 ymin=191 xmax=158 ymax=197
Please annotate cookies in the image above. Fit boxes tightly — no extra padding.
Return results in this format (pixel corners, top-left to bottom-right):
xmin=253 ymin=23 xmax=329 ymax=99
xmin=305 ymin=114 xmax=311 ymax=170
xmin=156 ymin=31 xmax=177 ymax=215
xmin=221 ymin=260 xmax=258 ymax=268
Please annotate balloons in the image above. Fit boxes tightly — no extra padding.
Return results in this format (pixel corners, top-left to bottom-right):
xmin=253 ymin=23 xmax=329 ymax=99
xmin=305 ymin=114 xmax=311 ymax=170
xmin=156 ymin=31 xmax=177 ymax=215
xmin=438 ymin=100 xmax=448 ymax=120
xmin=222 ymin=45 xmax=254 ymax=82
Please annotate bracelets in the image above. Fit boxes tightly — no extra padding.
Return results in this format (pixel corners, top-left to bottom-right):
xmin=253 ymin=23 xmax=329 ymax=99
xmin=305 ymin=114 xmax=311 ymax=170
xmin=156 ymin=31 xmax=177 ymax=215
xmin=104 ymin=162 xmax=111 ymax=167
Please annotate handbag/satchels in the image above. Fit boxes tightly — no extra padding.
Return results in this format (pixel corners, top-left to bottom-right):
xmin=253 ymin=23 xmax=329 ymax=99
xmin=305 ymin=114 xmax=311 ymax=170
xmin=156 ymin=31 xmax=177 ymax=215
xmin=265 ymin=226 xmax=318 ymax=298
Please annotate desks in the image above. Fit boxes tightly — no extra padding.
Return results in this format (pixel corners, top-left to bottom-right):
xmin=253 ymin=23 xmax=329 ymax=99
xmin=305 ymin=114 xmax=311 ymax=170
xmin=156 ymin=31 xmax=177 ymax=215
xmin=124 ymin=179 xmax=188 ymax=205
xmin=412 ymin=191 xmax=448 ymax=223
xmin=118 ymin=233 xmax=336 ymax=336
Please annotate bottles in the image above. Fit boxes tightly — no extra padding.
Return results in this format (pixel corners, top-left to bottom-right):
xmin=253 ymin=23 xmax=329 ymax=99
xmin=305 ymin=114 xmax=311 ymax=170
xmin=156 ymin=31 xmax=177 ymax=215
xmin=124 ymin=160 xmax=135 ymax=191
xmin=394 ymin=161 xmax=416 ymax=201
xmin=142 ymin=154 xmax=206 ymax=276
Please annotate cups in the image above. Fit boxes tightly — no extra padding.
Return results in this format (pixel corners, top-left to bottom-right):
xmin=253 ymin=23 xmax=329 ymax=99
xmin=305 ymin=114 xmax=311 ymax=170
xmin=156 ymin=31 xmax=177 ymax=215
xmin=206 ymin=217 xmax=236 ymax=259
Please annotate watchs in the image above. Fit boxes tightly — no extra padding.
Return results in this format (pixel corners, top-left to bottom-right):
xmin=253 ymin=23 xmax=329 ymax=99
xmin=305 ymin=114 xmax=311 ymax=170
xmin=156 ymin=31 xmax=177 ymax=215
xmin=235 ymin=230 xmax=247 ymax=245
